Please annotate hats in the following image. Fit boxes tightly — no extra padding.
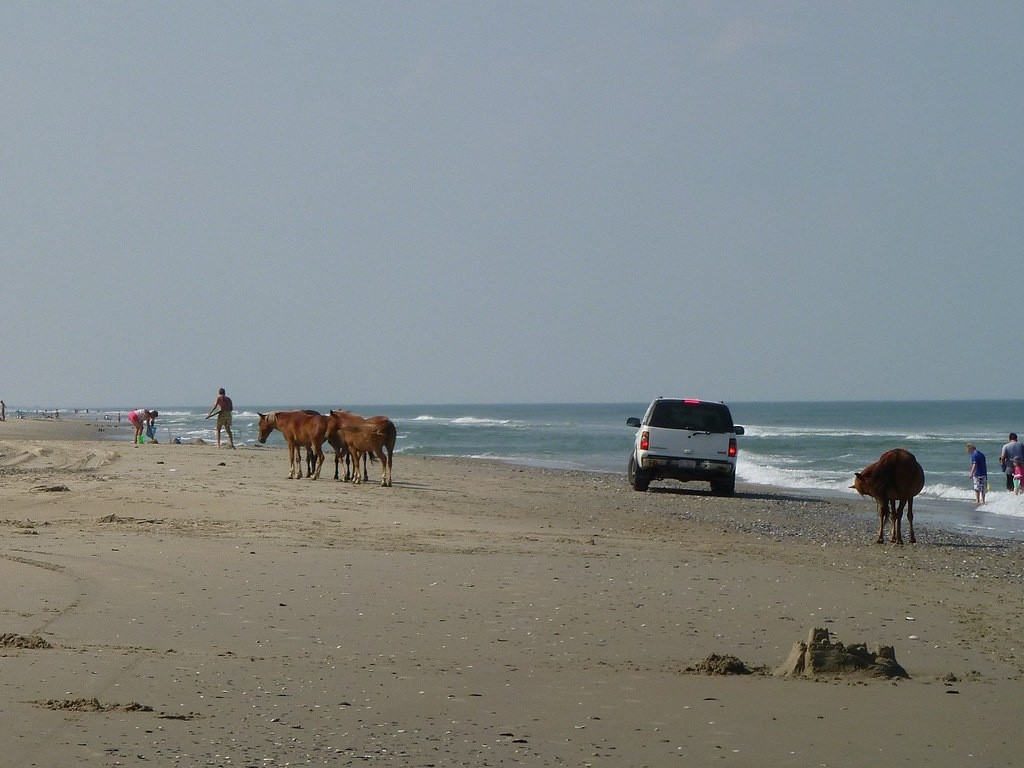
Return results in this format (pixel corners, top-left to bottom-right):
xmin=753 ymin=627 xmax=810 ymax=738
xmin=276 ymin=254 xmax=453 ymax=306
xmin=1010 ymin=456 xmax=1024 ymax=463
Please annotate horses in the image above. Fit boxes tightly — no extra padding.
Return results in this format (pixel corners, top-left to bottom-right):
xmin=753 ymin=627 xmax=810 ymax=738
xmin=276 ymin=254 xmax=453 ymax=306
xmin=324 ymin=409 xmax=397 ymax=488
xmin=257 ymin=409 xmax=347 ymax=481
xmin=847 ymin=449 xmax=925 ymax=545
xmin=298 ymin=409 xmax=381 ymax=482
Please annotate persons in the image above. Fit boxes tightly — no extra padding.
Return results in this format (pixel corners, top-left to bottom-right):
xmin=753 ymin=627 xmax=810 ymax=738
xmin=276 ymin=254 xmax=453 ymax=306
xmin=1000 ymin=433 xmax=1024 ymax=492
xmin=967 ymin=444 xmax=987 ymax=503
xmin=1009 ymin=456 xmax=1024 ymax=495
xmin=0 ymin=400 xmax=7 ymax=421
xmin=128 ymin=408 xmax=158 ymax=443
xmin=208 ymin=388 xmax=236 ymax=448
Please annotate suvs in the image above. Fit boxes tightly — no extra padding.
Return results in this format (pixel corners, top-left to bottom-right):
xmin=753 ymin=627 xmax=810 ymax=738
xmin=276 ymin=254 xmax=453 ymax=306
xmin=626 ymin=396 xmax=745 ymax=496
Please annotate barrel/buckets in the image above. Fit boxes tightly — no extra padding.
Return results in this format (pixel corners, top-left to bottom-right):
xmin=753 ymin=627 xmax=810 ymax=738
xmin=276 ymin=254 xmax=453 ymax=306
xmin=1013 ymin=476 xmax=1021 ymax=487
xmin=146 ymin=425 xmax=156 ymax=438
xmin=137 ymin=435 xmax=143 ymax=444
